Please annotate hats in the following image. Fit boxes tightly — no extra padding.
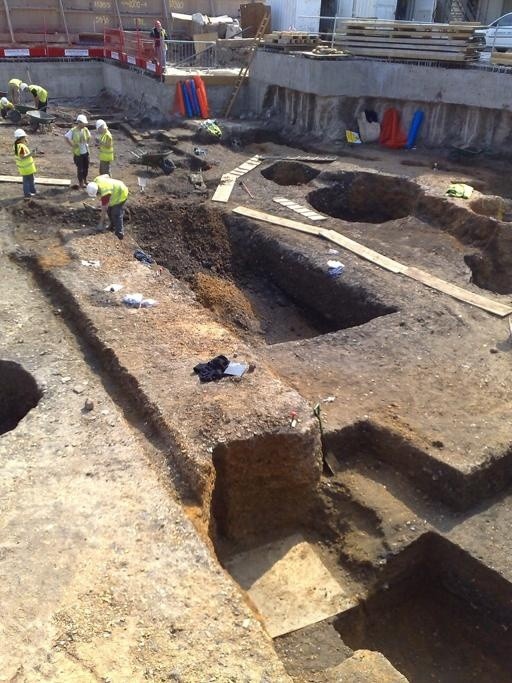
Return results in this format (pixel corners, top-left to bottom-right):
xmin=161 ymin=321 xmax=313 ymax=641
xmin=1 ymin=97 xmax=8 ymax=106
xmin=14 ymin=129 xmax=29 ymax=137
xmin=95 ymin=119 xmax=105 ymax=131
xmin=21 ymin=83 xmax=27 ymax=91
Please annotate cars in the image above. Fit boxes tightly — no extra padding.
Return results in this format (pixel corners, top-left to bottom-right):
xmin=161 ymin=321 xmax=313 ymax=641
xmin=470 ymin=11 xmax=512 ymax=52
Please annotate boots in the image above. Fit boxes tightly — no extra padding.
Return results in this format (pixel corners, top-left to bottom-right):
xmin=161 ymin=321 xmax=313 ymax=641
xmin=79 ymin=179 xmax=88 ymax=188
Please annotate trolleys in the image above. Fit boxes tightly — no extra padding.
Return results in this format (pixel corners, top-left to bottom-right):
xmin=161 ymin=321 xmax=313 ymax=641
xmin=8 ymin=105 xmax=57 ymax=134
xmin=125 ymin=148 xmax=176 ymax=178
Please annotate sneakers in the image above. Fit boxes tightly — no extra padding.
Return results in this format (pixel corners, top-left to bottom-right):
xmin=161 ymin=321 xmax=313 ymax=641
xmin=106 ymin=223 xmax=123 ymax=241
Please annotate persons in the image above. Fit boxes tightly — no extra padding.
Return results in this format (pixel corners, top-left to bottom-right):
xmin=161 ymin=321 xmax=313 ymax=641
xmin=149 ymin=19 xmax=169 ymax=73
xmin=14 ymin=128 xmax=41 ymax=195
xmin=93 ymin=118 xmax=113 ymax=178
xmin=64 ymin=114 xmax=92 ymax=191
xmin=20 ymin=82 xmax=49 ymax=112
xmin=87 ymin=172 xmax=128 ymax=239
xmin=0 ymin=96 xmax=16 ymax=118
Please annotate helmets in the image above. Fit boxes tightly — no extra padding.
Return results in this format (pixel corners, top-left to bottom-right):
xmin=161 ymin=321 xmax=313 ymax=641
xmin=86 ymin=182 xmax=98 ymax=197
xmin=77 ymin=115 xmax=88 ymax=124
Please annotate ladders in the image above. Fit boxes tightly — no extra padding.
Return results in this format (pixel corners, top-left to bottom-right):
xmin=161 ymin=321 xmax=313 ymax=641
xmin=221 ymin=12 xmax=271 ymax=120
